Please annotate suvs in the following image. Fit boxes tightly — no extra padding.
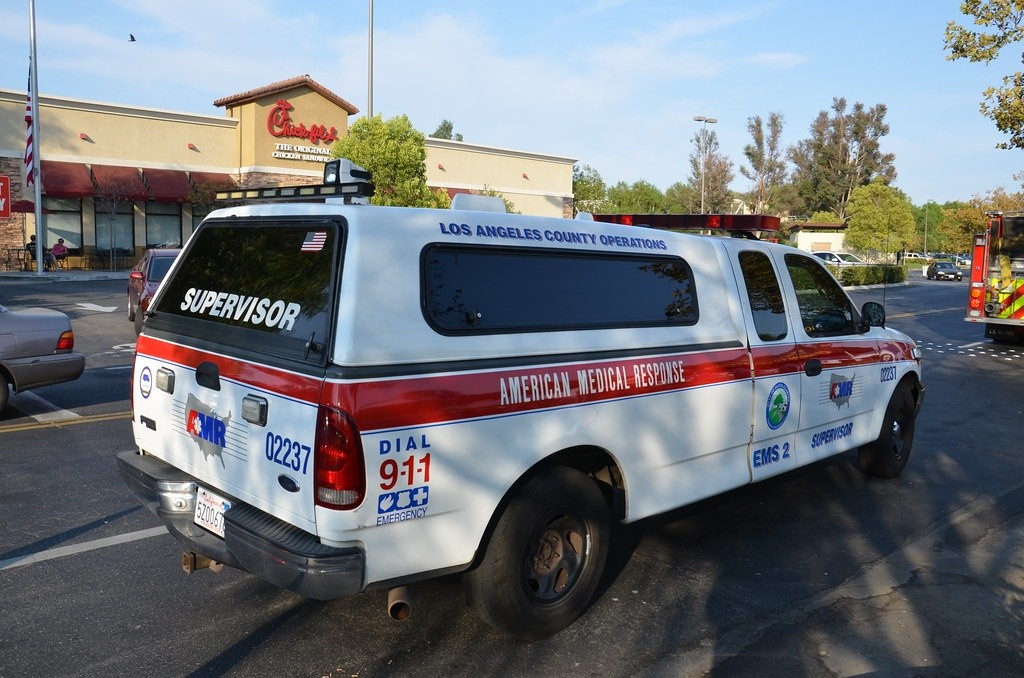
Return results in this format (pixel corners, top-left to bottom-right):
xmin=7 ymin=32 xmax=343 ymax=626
xmin=115 ymin=159 xmax=921 ymax=646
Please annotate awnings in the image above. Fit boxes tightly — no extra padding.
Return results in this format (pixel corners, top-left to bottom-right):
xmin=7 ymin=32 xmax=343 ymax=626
xmin=142 ymin=168 xmax=194 ymax=202
xmin=90 ymin=164 xmax=149 ymax=202
xmin=40 ymin=160 xmax=96 ymax=200
xmin=189 ymin=171 xmax=234 ymax=191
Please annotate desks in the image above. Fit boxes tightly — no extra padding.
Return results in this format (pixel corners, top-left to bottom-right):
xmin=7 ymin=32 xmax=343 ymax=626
xmin=2 ymin=249 xmax=33 ymax=272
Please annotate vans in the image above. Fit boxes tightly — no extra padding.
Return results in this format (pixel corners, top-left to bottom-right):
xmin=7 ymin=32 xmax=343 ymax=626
xmin=905 ymin=253 xmax=923 ymax=258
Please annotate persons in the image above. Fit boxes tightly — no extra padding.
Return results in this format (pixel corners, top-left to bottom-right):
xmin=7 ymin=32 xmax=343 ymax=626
xmin=46 ymin=238 xmax=68 ymax=268
xmin=26 ymin=235 xmax=60 ymax=272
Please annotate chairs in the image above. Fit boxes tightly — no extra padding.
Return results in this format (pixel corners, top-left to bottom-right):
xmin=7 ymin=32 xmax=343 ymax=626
xmin=3 ymin=246 xmax=70 ymax=273
xmin=79 ymin=244 xmax=131 ymax=274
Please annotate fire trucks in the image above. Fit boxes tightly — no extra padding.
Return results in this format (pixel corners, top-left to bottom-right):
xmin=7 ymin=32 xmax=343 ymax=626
xmin=964 ymin=210 xmax=1024 ymax=343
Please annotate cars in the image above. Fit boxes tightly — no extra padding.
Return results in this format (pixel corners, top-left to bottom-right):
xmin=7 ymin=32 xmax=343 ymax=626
xmin=810 ymin=251 xmax=866 ymax=266
xmin=0 ymin=303 xmax=85 ymax=415
xmin=927 ymin=262 xmax=962 ymax=281
xmin=126 ymin=249 xmax=184 ymax=338
xmin=922 ymin=253 xmax=972 ymax=265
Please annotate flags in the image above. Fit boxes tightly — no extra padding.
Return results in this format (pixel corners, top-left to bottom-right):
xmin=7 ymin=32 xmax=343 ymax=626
xmin=23 ymin=56 xmax=36 ymax=192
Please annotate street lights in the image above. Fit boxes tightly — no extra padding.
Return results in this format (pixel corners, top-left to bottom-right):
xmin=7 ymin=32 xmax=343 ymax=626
xmin=693 ymin=115 xmax=717 ymax=235
